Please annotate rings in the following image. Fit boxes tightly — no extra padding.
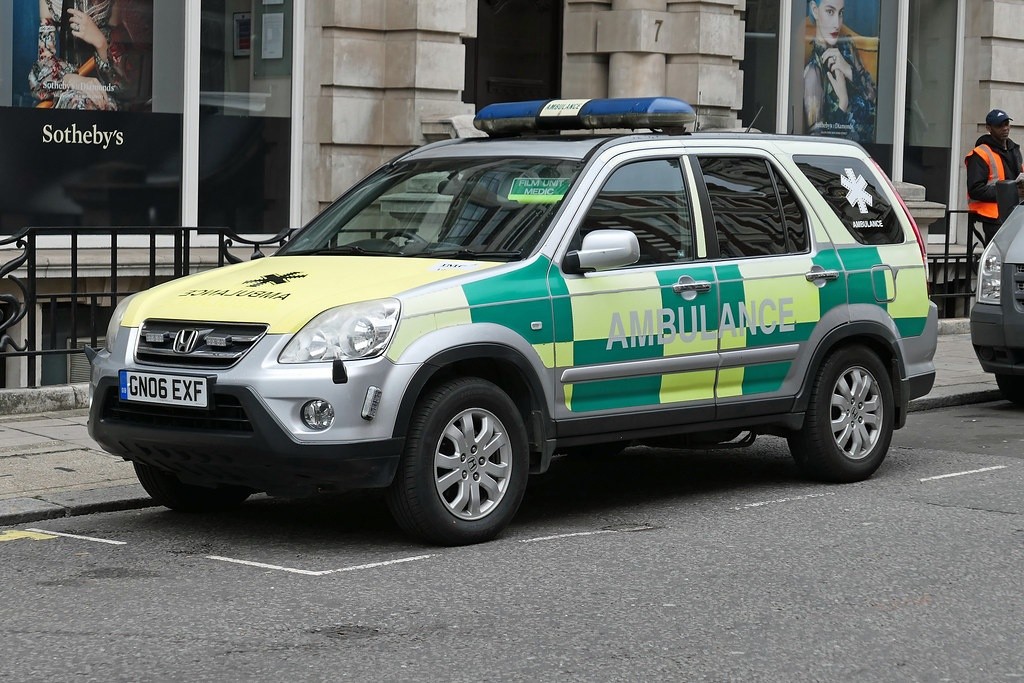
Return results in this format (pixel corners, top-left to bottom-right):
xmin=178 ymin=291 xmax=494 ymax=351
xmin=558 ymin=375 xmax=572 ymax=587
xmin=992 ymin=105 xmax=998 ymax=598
xmin=74 ymin=23 xmax=80 ymax=31
xmin=833 ymin=56 xmax=835 ymax=62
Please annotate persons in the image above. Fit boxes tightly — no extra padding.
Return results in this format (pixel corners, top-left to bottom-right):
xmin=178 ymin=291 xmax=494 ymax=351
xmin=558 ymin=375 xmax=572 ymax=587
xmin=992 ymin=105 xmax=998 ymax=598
xmin=964 ymin=109 xmax=1024 ymax=248
xmin=804 ymin=0 xmax=877 ymax=143
xmin=22 ymin=1 xmax=153 ymax=111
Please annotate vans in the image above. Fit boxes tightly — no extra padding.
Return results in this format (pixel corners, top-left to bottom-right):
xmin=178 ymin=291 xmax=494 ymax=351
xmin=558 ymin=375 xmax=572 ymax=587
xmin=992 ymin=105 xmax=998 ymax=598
xmin=969 ymin=198 xmax=1024 ymax=404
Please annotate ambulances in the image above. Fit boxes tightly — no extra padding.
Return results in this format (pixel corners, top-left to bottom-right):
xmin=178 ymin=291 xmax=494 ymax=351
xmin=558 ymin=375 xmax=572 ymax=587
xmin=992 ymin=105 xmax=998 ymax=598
xmin=86 ymin=96 xmax=940 ymax=548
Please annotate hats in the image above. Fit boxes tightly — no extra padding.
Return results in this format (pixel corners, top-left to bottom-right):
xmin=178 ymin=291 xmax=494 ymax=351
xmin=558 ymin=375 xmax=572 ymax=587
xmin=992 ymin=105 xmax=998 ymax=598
xmin=986 ymin=110 xmax=1013 ymax=126
